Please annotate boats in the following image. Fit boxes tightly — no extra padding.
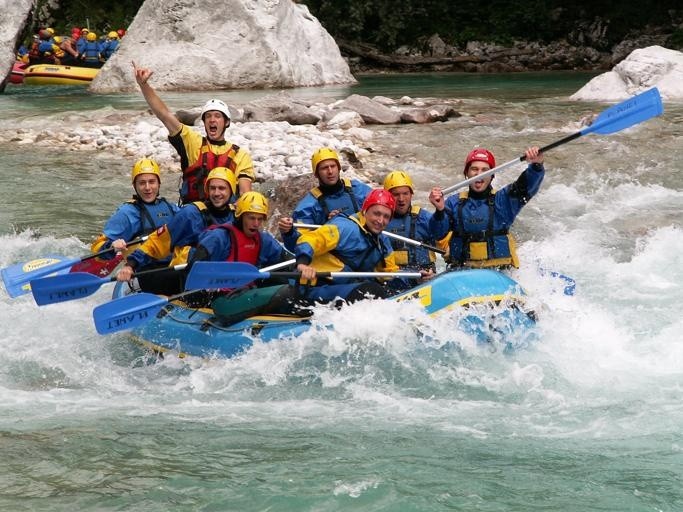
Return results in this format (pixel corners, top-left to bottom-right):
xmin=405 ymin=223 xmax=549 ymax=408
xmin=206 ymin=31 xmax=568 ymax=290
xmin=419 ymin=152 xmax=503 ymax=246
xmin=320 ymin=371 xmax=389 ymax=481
xmin=23 ymin=64 xmax=99 ymax=84
xmin=112 ymin=266 xmax=564 ymax=369
xmin=10 ymin=60 xmax=29 ymax=83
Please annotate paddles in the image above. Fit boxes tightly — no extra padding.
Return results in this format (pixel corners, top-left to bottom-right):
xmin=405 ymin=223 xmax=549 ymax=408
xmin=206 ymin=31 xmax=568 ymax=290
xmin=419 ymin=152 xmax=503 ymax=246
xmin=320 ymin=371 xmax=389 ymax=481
xmin=184 ymin=261 xmax=422 ymax=289
xmin=0 ymin=235 xmax=148 ymax=299
xmin=93 ymin=259 xmax=297 ymax=336
xmin=29 ymin=262 xmax=188 ymax=307
xmin=441 ymin=86 xmax=664 ymax=195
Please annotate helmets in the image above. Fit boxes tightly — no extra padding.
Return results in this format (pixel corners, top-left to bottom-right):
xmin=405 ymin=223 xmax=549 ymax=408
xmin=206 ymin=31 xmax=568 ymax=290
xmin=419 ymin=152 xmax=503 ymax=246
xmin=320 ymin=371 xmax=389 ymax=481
xmin=46 ymin=28 xmax=54 ymax=33
xmin=384 ymin=172 xmax=414 ymax=194
xmin=87 ymin=33 xmax=96 ymax=40
xmin=202 ymin=99 xmax=231 ymax=121
xmin=82 ymin=29 xmax=89 ymax=33
xmin=312 ymin=149 xmax=341 ymax=175
xmin=109 ymin=31 xmax=118 ymax=39
xmin=117 ymin=30 xmax=125 ymax=36
xmin=133 ymin=159 xmax=160 ymax=184
xmin=363 ymin=189 xmax=396 ymax=214
xmin=72 ymin=33 xmax=79 ymax=40
xmin=42 ymin=31 xmax=52 ymax=39
xmin=206 ymin=168 xmax=236 ymax=195
xmin=464 ymin=150 xmax=495 ymax=168
xmin=236 ymin=191 xmax=268 ymax=219
xmin=73 ymin=29 xmax=80 ymax=35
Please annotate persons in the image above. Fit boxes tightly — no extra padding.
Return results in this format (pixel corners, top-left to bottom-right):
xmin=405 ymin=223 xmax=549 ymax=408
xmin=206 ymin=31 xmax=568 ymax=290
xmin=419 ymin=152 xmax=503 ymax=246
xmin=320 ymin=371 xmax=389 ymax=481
xmin=132 ymin=60 xmax=254 ymax=201
xmin=295 ymin=189 xmax=433 ymax=296
xmin=77 ymin=33 xmax=104 ymax=68
xmin=382 ymin=172 xmax=452 ymax=282
xmin=28 ymin=30 xmax=58 ymax=63
xmin=61 ymin=27 xmax=83 ymax=63
xmin=113 ymin=166 xmax=236 ymax=292
xmin=116 ymin=29 xmax=126 ymax=38
xmin=101 ymin=31 xmax=120 ymax=61
xmin=79 ymin=29 xmax=90 ymax=53
xmin=429 ymin=147 xmax=544 ymax=291
xmin=188 ymin=192 xmax=313 ymax=315
xmin=43 ymin=28 xmax=62 ymax=56
xmin=90 ymin=157 xmax=183 ymax=261
xmin=277 ymin=145 xmax=380 ymax=253
